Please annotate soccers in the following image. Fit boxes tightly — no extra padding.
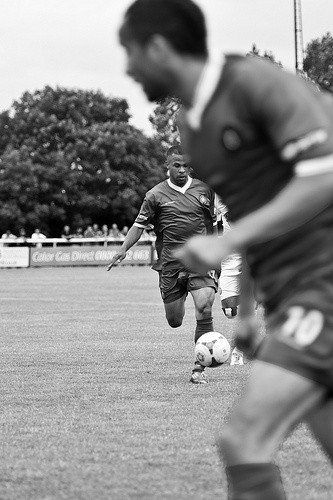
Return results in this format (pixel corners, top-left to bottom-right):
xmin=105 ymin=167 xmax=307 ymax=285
xmin=195 ymin=331 xmax=230 ymax=368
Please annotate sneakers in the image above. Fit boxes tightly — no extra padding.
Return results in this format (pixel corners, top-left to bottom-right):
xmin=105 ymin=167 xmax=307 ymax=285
xmin=191 ymin=371 xmax=209 ymax=383
xmin=230 ymin=349 xmax=244 ymax=365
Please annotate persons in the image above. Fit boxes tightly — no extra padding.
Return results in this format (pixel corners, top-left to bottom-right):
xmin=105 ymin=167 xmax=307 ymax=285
xmin=213 ymin=191 xmax=255 ymax=367
xmin=119 ymin=0 xmax=333 ymax=500
xmin=107 ymin=145 xmax=219 ymax=384
